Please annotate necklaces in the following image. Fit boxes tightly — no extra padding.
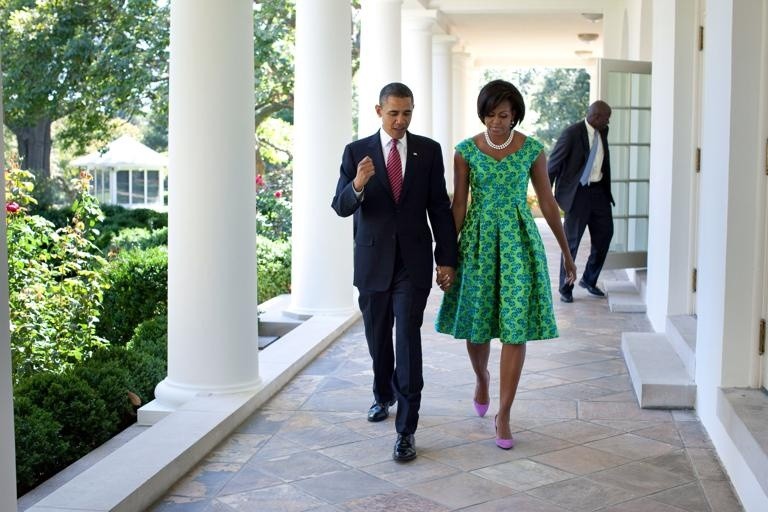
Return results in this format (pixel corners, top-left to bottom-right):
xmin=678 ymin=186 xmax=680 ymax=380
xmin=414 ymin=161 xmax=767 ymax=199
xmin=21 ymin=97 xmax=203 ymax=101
xmin=482 ymin=128 xmax=516 ymax=150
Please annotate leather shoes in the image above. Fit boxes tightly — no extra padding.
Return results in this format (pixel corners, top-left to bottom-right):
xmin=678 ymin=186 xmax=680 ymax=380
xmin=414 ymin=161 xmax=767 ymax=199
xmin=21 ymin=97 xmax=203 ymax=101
xmin=392 ymin=433 xmax=418 ymax=462
xmin=560 ymin=293 xmax=573 ymax=302
xmin=579 ymin=277 xmax=605 ymax=296
xmin=368 ymin=400 xmax=396 ymax=422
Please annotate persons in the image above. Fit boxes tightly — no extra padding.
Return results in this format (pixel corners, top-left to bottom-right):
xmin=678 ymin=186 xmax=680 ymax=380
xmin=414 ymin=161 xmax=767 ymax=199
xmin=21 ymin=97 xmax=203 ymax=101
xmin=547 ymin=100 xmax=618 ymax=303
xmin=433 ymin=79 xmax=581 ymax=451
xmin=329 ymin=82 xmax=461 ymax=462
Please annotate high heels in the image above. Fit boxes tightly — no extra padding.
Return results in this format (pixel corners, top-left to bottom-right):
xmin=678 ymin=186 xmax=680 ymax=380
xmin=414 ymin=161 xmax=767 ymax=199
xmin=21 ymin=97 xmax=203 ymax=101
xmin=495 ymin=414 xmax=514 ymax=450
xmin=474 ymin=371 xmax=490 ymax=417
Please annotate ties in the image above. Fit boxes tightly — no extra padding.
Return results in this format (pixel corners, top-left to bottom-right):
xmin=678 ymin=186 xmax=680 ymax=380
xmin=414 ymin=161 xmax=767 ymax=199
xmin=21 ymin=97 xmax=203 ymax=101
xmin=580 ymin=129 xmax=599 ymax=187
xmin=387 ymin=139 xmax=403 ymax=204
